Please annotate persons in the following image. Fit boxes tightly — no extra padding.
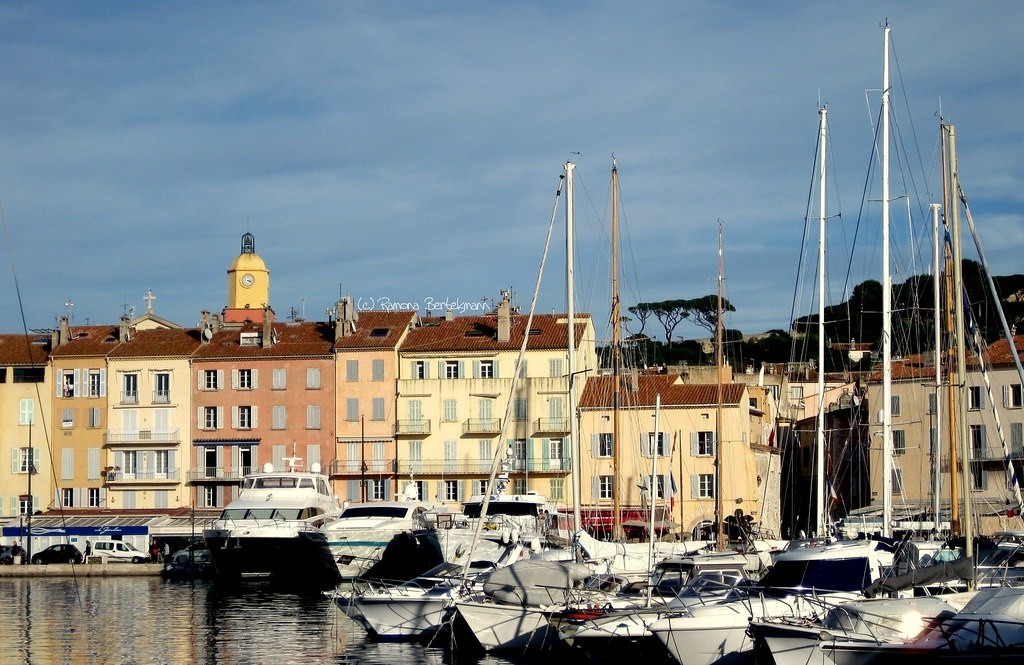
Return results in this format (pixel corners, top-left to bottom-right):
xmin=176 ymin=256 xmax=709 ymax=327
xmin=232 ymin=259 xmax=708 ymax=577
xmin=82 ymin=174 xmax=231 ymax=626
xmin=82 ymin=540 xmax=91 ymax=564
xmin=149 ymin=540 xmax=169 ymax=564
xmin=11 ymin=541 xmax=18 ymax=556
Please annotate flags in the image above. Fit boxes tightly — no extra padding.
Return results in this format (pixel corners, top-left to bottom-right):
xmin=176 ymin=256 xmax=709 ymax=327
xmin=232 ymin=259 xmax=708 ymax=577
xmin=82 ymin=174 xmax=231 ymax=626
xmin=826 ymin=474 xmax=840 ymax=505
xmin=669 ymin=470 xmax=674 ymax=513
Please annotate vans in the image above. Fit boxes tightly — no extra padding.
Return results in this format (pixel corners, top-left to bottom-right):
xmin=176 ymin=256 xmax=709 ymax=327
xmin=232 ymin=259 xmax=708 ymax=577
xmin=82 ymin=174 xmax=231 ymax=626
xmin=95 ymin=541 xmax=151 ymax=565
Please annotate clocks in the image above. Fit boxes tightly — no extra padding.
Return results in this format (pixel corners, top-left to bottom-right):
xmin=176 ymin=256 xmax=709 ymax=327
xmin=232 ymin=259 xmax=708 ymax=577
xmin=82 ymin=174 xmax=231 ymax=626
xmin=242 ymin=275 xmax=254 ymax=286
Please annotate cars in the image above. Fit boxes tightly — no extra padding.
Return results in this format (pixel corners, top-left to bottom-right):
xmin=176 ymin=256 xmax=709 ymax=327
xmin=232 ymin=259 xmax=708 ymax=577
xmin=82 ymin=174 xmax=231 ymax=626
xmin=0 ymin=545 xmax=27 ymax=566
xmin=172 ymin=541 xmax=207 ymax=565
xmin=31 ymin=544 xmax=84 ymax=566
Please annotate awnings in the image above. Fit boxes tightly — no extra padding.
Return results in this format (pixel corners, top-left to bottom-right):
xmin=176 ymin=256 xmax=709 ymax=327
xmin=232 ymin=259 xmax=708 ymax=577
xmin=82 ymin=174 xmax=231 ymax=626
xmin=2 ymin=516 xmax=166 ymax=536
xmin=849 ymin=503 xmax=1019 ymax=516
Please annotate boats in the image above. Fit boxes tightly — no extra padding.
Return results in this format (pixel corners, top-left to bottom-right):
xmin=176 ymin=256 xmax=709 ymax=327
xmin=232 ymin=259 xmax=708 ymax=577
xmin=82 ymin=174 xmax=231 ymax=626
xmin=202 ymin=456 xmax=343 ymax=579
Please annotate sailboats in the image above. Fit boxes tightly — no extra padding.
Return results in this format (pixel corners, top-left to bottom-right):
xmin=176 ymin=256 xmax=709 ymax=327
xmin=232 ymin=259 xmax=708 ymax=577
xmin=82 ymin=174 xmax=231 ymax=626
xmin=323 ymin=15 xmax=1024 ymax=664
